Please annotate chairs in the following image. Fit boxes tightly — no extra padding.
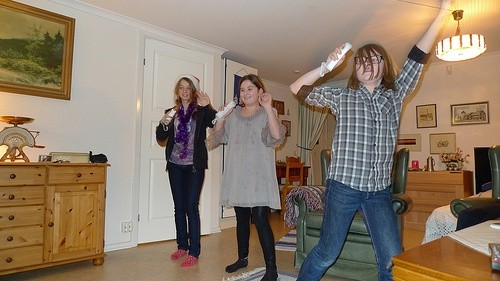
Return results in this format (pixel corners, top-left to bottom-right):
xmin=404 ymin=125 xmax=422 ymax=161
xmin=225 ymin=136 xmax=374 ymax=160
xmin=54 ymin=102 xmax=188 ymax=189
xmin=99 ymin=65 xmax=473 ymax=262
xmin=279 ymin=162 xmax=306 ymax=220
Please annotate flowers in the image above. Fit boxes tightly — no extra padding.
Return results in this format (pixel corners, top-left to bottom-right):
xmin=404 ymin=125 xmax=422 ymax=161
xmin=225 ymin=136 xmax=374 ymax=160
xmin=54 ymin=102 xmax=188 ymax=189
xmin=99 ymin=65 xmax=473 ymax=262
xmin=437 ymin=147 xmax=470 ymax=165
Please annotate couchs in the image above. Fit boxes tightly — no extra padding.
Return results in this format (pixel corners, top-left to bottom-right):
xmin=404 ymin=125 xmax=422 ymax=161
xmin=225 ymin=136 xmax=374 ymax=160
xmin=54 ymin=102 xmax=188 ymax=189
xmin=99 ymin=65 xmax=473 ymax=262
xmin=283 ymin=147 xmax=413 ymax=281
xmin=449 ymin=143 xmax=500 ymax=217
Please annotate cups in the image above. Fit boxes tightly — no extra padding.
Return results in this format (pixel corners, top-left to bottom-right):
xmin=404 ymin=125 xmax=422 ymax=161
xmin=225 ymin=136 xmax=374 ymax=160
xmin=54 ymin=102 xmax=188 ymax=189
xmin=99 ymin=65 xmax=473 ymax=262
xmin=412 ymin=160 xmax=419 ymax=169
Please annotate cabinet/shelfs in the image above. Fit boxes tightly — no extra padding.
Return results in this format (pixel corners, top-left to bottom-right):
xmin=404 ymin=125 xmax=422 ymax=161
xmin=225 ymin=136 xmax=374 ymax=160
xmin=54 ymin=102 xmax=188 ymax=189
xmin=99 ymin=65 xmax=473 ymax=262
xmin=403 ymin=171 xmax=474 ymax=231
xmin=0 ymin=161 xmax=111 ymax=276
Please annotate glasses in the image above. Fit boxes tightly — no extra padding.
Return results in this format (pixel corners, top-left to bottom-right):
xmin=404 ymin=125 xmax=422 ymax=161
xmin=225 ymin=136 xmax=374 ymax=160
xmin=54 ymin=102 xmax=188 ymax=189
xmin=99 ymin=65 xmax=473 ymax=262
xmin=354 ymin=55 xmax=384 ymax=64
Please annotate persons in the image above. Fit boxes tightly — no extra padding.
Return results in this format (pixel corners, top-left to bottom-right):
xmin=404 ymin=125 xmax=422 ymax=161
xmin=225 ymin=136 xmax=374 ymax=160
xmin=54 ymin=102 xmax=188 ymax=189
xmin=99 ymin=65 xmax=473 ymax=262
xmin=205 ymin=74 xmax=287 ymax=281
xmin=289 ymin=0 xmax=451 ymax=281
xmin=156 ymin=77 xmax=217 ymax=267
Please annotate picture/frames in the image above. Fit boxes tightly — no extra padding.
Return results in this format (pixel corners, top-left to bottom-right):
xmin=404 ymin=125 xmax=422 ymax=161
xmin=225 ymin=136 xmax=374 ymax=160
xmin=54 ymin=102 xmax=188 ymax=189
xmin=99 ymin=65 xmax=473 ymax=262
xmin=272 ymin=99 xmax=292 ymax=136
xmin=396 ymin=134 xmax=421 ymax=152
xmin=416 ymin=104 xmax=437 ymax=128
xmin=429 ymin=132 xmax=457 ymax=154
xmin=450 ymin=101 xmax=489 ymax=126
xmin=0 ymin=0 xmax=76 ymax=100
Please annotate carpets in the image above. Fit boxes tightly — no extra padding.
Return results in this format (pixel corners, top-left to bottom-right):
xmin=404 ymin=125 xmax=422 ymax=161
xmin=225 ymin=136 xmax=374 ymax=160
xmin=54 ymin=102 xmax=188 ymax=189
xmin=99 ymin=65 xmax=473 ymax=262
xmin=223 ymin=267 xmax=300 ymax=281
xmin=274 ymin=229 xmax=297 ymax=252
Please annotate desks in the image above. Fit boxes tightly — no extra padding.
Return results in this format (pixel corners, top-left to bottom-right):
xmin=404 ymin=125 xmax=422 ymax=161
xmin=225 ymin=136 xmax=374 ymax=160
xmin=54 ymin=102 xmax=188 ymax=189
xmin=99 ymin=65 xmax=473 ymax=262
xmin=391 ymin=217 xmax=500 ymax=281
xmin=278 ymin=165 xmax=311 ymax=186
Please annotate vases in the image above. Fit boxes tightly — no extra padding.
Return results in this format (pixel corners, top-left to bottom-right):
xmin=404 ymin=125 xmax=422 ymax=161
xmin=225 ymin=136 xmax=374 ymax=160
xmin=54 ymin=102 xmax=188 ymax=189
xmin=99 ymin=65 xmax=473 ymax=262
xmin=444 ymin=161 xmax=463 ymax=170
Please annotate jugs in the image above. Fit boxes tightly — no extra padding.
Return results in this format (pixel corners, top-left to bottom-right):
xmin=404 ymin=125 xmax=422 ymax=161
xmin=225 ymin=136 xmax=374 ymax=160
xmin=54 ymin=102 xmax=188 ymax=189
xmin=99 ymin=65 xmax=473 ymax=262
xmin=427 ymin=156 xmax=436 ymax=171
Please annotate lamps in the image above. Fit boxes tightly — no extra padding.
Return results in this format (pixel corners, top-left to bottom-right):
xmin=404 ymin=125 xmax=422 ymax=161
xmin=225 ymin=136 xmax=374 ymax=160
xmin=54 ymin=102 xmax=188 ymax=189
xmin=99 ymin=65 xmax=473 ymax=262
xmin=435 ymin=9 xmax=487 ymax=62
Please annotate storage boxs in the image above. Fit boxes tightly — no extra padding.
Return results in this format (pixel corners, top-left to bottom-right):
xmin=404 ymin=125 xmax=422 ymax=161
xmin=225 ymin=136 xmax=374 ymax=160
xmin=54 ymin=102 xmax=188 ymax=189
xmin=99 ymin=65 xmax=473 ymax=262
xmin=488 ymin=242 xmax=500 ymax=270
